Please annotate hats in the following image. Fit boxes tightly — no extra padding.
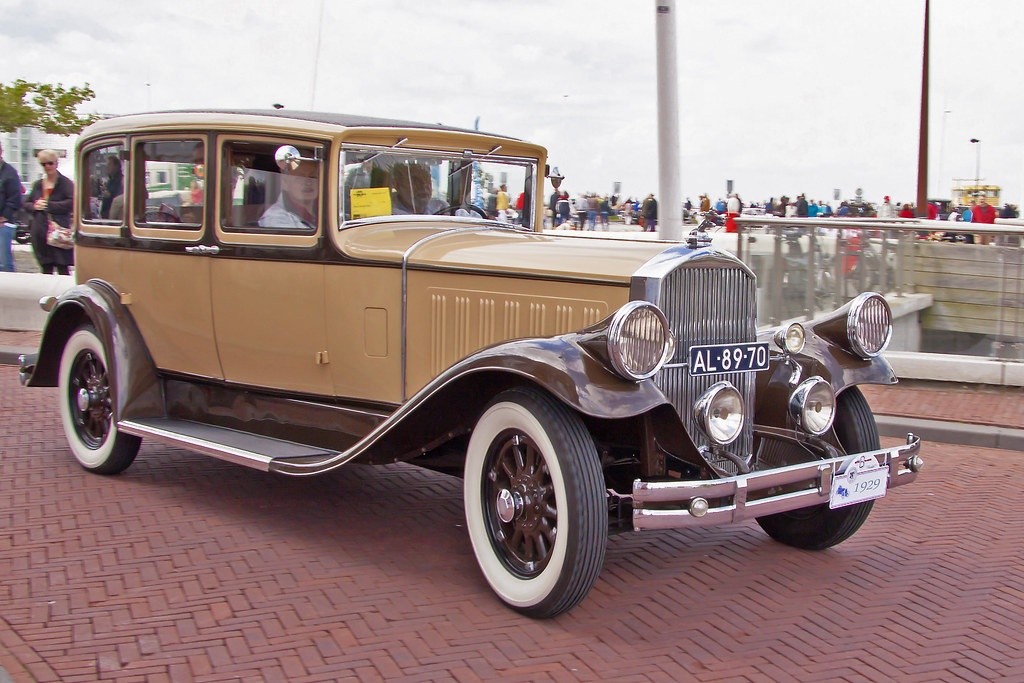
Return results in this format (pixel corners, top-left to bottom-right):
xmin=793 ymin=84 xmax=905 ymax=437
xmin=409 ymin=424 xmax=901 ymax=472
xmin=798 ymin=192 xmax=805 ymax=198
xmin=884 ymin=195 xmax=889 ymax=201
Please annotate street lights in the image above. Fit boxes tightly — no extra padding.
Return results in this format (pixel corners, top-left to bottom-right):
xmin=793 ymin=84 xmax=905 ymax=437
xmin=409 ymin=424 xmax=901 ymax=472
xmin=970 ymin=138 xmax=981 ymax=188
xmin=936 ymin=109 xmax=952 ymax=199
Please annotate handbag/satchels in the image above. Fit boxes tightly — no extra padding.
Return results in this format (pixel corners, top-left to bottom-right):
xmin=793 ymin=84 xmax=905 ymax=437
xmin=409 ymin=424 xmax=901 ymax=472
xmin=46 ymin=221 xmax=74 ymax=250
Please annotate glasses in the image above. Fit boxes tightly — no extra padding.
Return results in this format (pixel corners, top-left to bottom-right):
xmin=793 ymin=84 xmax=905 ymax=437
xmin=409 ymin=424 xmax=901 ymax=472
xmin=41 ymin=162 xmax=53 ymax=167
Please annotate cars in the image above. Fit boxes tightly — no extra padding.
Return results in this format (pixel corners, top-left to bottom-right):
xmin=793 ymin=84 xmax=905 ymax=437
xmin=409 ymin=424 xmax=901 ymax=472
xmin=17 ymin=106 xmax=924 ymax=620
xmin=144 ymin=160 xmax=205 ymax=225
xmin=16 ymin=188 xmax=32 ymax=245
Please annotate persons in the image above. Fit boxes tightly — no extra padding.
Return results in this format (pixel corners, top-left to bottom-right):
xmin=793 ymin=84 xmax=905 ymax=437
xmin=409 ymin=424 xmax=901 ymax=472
xmin=575 ymin=192 xmax=611 ymax=231
xmin=896 ymin=202 xmax=919 ymax=218
xmin=256 ymin=150 xmax=319 ymax=228
xmin=624 ymin=194 xmax=658 ymax=232
xmin=489 ymin=184 xmax=509 ymax=223
xmin=928 ymin=192 xmax=996 ymax=223
xmin=0 ymin=142 xmax=23 ymax=272
xmin=687 ymin=201 xmax=691 ymax=210
xmin=550 ymin=190 xmax=570 ymax=229
xmin=1001 ymin=203 xmax=1017 ymax=218
xmin=750 ymin=203 xmax=759 ymax=208
xmin=766 ymin=196 xmax=831 ymax=234
xmin=700 ymin=194 xmax=710 ymax=211
xmin=392 ymin=162 xmax=497 ymax=220
xmin=716 ymin=193 xmax=742 ymax=216
xmin=192 ymin=181 xmax=203 ymax=204
xmin=23 ymin=149 xmax=75 ymax=275
xmin=91 ymin=156 xmax=124 ymax=218
xmin=837 ymin=201 xmax=877 ymax=218
xmin=878 ymin=195 xmax=896 ymax=218
